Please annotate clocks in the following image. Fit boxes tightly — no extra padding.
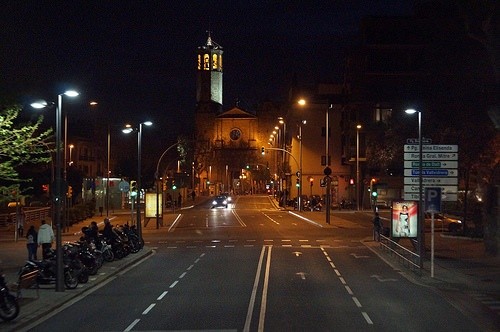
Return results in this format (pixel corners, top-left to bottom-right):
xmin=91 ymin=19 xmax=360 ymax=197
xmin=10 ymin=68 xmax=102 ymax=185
xmin=229 ymin=128 xmax=242 ymax=141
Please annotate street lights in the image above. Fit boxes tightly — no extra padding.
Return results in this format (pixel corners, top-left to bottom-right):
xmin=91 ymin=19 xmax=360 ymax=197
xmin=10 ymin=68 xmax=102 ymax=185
xmin=267 ymin=117 xmax=288 ymax=208
xmin=120 ymin=120 xmax=155 ymax=239
xmin=356 ymin=124 xmax=363 ymax=211
xmin=404 ymin=107 xmax=426 ymax=271
xmin=325 ymin=103 xmax=334 ymax=224
xmin=27 ymin=88 xmax=82 ymax=292
xmin=69 ymin=144 xmax=75 ymax=163
xmin=89 ymin=101 xmax=112 ymax=178
xmin=298 ymin=118 xmax=308 ymax=214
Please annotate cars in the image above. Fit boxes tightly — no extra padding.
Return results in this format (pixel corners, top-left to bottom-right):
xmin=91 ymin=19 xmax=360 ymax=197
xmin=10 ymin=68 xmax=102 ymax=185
xmin=211 ymin=192 xmax=232 ymax=208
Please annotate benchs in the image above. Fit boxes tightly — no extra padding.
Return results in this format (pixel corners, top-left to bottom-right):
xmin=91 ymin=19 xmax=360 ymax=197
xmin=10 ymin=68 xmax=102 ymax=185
xmin=8 ymin=262 xmax=42 ymax=301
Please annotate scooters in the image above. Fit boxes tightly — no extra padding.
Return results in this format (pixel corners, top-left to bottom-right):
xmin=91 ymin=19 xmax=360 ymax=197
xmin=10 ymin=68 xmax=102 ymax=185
xmin=18 ymin=220 xmax=147 ymax=290
xmin=0 ymin=268 xmax=20 ymax=323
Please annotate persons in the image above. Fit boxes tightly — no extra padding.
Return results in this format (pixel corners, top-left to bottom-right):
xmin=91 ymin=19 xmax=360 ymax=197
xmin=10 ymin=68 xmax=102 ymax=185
xmin=341 ymin=199 xmax=345 ymax=208
xmin=89 ymin=198 xmax=96 ymax=213
xmin=26 ymin=225 xmax=38 ymax=262
xmin=18 ymin=225 xmax=24 ymax=238
xmin=38 ymin=219 xmax=54 ymax=259
xmin=311 ymin=196 xmax=318 ymax=206
xmin=0 ymin=266 xmax=12 ymax=315
xmin=191 ymin=190 xmax=195 ymax=200
xmin=178 ymin=192 xmax=182 ymax=207
xmin=471 ymin=203 xmax=484 ymax=241
xmin=80 ymin=218 xmax=113 ymax=243
xmin=396 ymin=206 xmax=410 ymax=237
xmin=370 ymin=212 xmax=384 ymax=242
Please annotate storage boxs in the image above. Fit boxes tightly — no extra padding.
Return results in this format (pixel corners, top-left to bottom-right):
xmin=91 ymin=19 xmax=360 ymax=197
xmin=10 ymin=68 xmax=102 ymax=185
xmin=20 ymin=265 xmax=43 ymax=290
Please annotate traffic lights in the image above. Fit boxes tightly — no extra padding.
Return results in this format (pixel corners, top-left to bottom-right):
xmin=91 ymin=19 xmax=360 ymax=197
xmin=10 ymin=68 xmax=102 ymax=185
xmin=261 ymin=146 xmax=267 ymax=158
xmin=372 ymin=183 xmax=378 ymax=201
